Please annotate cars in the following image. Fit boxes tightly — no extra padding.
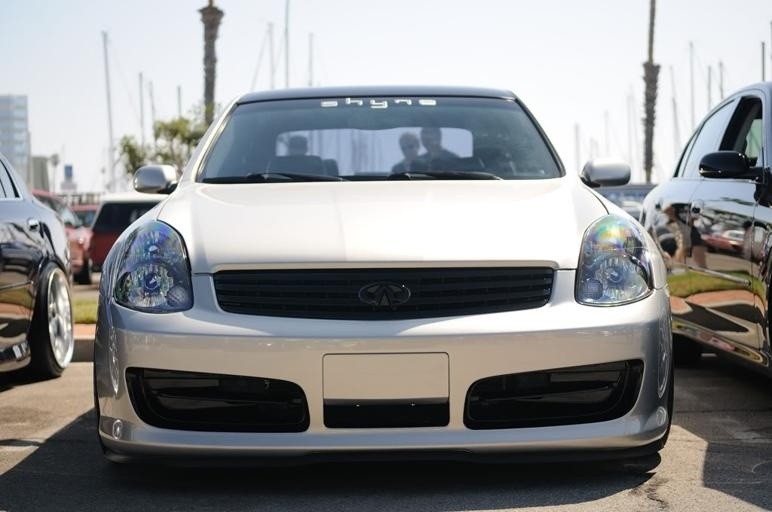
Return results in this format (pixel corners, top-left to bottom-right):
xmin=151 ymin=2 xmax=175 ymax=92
xmin=698 ymin=220 xmax=769 ymax=261
xmin=1 ymin=149 xmax=81 ymax=397
xmin=29 ymin=186 xmax=169 ymax=286
xmin=640 ymin=79 xmax=772 ymax=377
xmin=87 ymin=79 xmax=691 ymax=475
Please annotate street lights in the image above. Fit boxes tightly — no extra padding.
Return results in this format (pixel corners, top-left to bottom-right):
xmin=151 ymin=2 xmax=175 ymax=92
xmin=195 ymin=0 xmax=225 ymax=126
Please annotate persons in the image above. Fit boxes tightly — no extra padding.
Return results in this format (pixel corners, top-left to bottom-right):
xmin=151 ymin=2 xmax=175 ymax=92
xmin=287 ymin=136 xmax=308 ymax=156
xmin=392 ymin=132 xmax=426 ymax=172
xmin=411 ymin=123 xmax=463 ymax=171
